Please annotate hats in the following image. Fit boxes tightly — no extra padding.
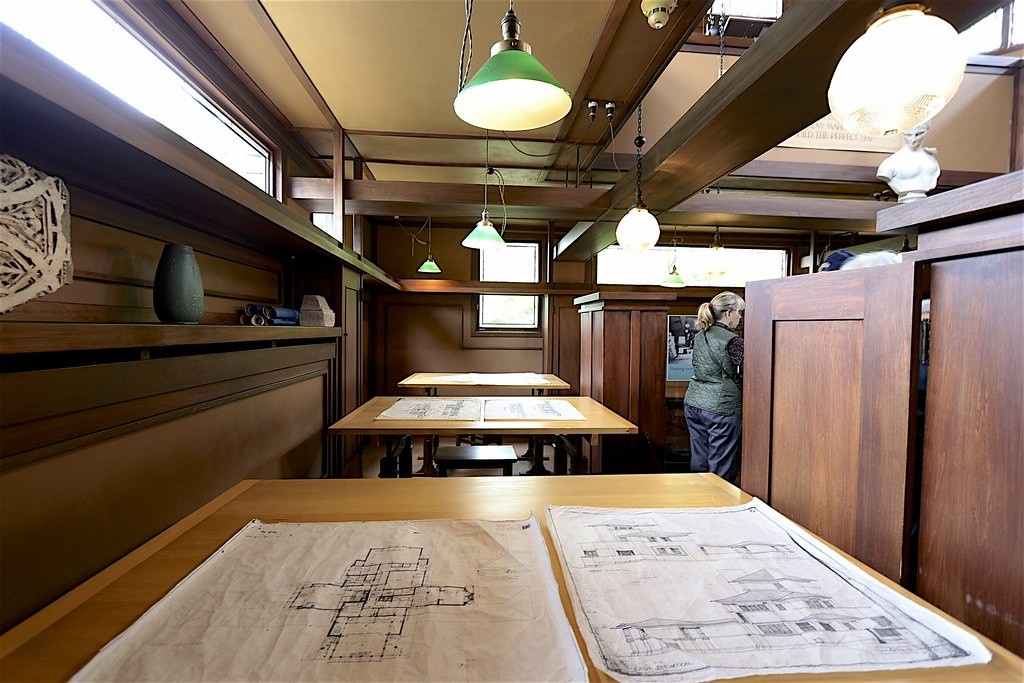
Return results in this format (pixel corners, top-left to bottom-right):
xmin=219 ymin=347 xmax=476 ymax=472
xmin=821 ymin=250 xmax=854 ymax=271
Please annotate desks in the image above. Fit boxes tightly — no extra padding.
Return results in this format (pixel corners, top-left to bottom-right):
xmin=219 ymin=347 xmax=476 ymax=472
xmin=0 ymin=473 xmax=1023 ymax=683
xmin=399 ymin=373 xmax=571 ymax=460
xmin=326 ymin=395 xmax=640 ymax=475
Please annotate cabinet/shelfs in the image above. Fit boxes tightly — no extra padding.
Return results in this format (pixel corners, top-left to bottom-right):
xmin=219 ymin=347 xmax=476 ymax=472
xmin=574 ymin=292 xmax=747 ymax=473
xmin=0 ymin=21 xmax=400 ymax=632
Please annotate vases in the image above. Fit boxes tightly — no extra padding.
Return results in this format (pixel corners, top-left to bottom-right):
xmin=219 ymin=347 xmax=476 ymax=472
xmin=154 ymin=241 xmax=204 ymax=324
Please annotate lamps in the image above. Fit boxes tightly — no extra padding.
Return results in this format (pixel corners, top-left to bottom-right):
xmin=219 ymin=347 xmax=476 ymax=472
xmin=615 ymin=103 xmax=660 ymax=248
xmin=462 ymin=172 xmax=505 ymax=248
xmin=418 ymin=217 xmax=442 ymax=272
xmin=661 ymin=226 xmax=685 ymax=286
xmin=453 ymin=0 xmax=572 ymax=131
xmin=826 ymin=1 xmax=964 ymax=140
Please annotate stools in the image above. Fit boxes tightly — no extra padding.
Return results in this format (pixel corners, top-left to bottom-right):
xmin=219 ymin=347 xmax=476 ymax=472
xmin=434 ymin=445 xmax=517 ymax=476
xmin=455 ymin=432 xmax=502 ymax=446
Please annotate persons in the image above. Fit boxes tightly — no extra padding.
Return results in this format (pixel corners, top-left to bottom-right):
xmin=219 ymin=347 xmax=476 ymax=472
xmin=877 ymin=119 xmax=941 ymax=205
xmin=683 ymin=291 xmax=746 ymax=484
